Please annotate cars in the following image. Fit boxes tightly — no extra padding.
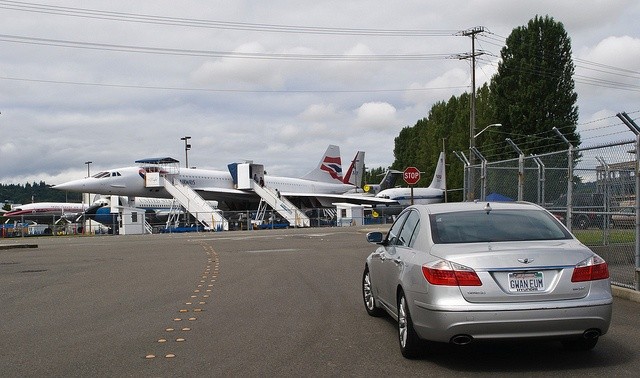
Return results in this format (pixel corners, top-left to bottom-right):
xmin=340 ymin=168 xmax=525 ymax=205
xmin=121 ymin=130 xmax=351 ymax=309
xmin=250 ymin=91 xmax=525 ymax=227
xmin=362 ymin=199 xmax=613 ymax=357
xmin=609 ymin=206 xmax=636 ymax=228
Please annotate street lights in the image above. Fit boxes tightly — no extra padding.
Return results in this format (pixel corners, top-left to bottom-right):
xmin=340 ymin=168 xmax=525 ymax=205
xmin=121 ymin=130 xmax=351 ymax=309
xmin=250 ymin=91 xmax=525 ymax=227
xmin=85 ymin=161 xmax=92 ymax=233
xmin=181 ymin=136 xmax=191 ymax=168
xmin=468 ymin=123 xmax=502 ymax=199
xmin=442 ymin=137 xmax=447 ymax=202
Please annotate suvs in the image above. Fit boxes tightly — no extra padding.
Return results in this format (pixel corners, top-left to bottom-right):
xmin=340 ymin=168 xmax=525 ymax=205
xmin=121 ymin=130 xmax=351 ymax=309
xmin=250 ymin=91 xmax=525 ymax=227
xmin=548 ymin=193 xmax=613 ymax=229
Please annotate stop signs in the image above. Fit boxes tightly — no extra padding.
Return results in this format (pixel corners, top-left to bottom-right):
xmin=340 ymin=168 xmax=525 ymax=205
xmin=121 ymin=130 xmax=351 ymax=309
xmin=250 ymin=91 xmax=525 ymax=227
xmin=403 ymin=166 xmax=421 ymax=184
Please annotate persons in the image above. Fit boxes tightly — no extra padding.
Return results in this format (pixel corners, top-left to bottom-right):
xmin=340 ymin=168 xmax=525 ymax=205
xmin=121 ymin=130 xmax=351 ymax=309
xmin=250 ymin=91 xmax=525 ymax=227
xmin=259 ymin=176 xmax=264 ymax=188
xmin=275 ymin=189 xmax=281 ymax=199
xmin=254 ymin=174 xmax=259 ymax=183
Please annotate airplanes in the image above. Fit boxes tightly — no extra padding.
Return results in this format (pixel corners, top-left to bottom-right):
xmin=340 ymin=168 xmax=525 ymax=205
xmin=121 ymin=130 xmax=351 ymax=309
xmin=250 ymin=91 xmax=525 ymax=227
xmin=375 ymin=151 xmax=445 ymax=214
xmin=75 ymin=197 xmax=219 ymax=227
xmin=48 ymin=145 xmax=365 ymax=230
xmin=366 ymin=169 xmax=425 ymax=193
xmin=3 ymin=201 xmax=83 ymax=217
